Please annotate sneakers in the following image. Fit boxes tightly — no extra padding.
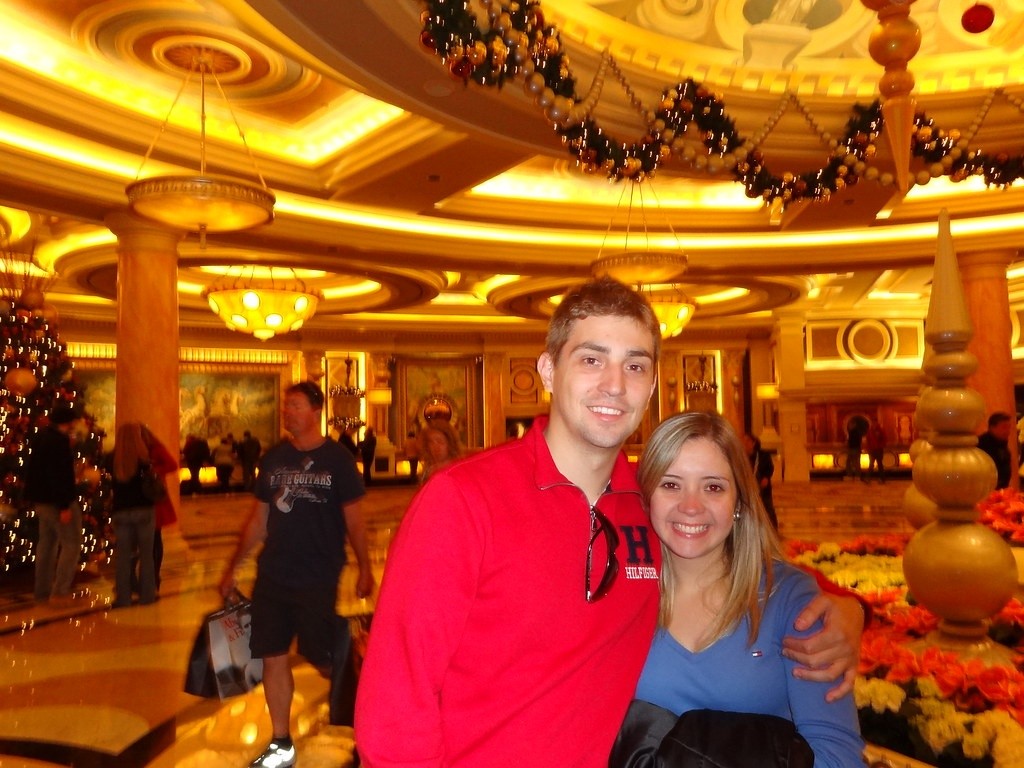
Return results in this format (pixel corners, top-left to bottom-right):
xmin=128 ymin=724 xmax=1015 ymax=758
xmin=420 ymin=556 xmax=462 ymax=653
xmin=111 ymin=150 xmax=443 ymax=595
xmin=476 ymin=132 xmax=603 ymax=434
xmin=248 ymin=739 xmax=297 ymax=768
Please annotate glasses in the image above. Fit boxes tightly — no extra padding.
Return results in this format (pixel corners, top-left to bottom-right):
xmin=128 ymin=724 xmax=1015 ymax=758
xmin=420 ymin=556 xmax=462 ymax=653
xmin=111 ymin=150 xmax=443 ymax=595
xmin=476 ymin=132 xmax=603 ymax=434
xmin=584 ymin=504 xmax=621 ymax=603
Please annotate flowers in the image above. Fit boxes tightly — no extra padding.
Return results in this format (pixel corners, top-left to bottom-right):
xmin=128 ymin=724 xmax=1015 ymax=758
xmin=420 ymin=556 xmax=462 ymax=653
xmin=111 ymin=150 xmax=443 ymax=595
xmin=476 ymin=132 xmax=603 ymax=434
xmin=779 ymin=485 xmax=1024 ymax=768
xmin=685 ymin=380 xmax=715 ymax=394
xmin=330 ymin=382 xmax=367 ymax=399
xmin=327 ymin=415 xmax=365 ymax=434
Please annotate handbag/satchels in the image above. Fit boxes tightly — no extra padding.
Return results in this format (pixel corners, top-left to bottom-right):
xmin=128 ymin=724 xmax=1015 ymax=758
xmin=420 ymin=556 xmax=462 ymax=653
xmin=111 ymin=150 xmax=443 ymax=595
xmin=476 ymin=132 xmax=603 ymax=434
xmin=184 ymin=589 xmax=264 ymax=700
xmin=330 ymin=596 xmax=377 ymax=728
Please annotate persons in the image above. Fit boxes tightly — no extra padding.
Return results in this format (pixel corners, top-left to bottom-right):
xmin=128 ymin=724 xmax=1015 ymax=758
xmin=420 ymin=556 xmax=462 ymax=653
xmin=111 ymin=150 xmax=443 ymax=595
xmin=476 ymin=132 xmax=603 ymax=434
xmin=183 ymin=429 xmax=260 ymax=492
xmin=742 ymin=432 xmax=777 ymax=531
xmin=844 ymin=414 xmax=886 ymax=485
xmin=24 ymin=404 xmax=89 ymax=604
xmin=352 ymin=276 xmax=874 ymax=768
xmin=337 ymin=424 xmax=356 ymax=454
xmin=104 ymin=421 xmax=177 ymax=608
xmin=634 ymin=409 xmax=869 ymax=768
xmin=405 ymin=430 xmax=420 ymax=484
xmin=357 ymin=427 xmax=376 ymax=486
xmin=221 ymin=379 xmax=374 ymax=768
xmin=417 ymin=419 xmax=465 ymax=478
xmin=1016 ymin=411 xmax=1024 ymax=492
xmin=978 ymin=410 xmax=1012 ymax=491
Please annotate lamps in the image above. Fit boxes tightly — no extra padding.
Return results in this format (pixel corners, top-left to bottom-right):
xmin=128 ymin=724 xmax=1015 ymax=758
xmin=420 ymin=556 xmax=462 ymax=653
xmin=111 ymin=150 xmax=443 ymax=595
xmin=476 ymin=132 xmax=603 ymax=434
xmin=588 ymin=175 xmax=688 ymax=285
xmin=200 ymin=263 xmax=325 ymax=343
xmin=757 ymin=382 xmax=780 ymax=399
xmin=126 ymin=52 xmax=276 ymax=249
xmin=370 ymin=387 xmax=392 ymax=404
xmin=632 ymin=282 xmax=698 ymax=340
